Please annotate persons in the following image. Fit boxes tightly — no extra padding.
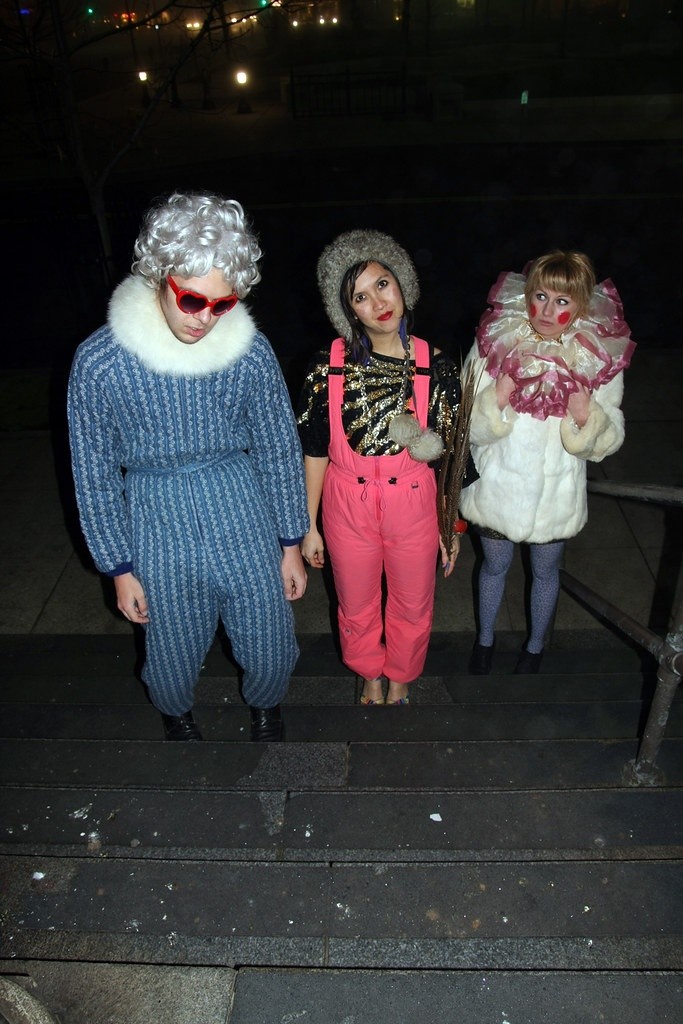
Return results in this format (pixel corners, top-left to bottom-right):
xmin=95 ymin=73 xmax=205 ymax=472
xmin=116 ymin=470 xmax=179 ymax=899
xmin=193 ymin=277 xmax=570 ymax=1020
xmin=461 ymin=251 xmax=637 ymax=676
xmin=294 ymin=230 xmax=479 ymax=705
xmin=65 ymin=192 xmax=308 ymax=740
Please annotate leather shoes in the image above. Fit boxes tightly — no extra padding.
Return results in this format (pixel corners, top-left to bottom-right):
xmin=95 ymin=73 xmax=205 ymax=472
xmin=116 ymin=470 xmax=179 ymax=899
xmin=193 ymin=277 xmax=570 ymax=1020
xmin=519 ymin=642 xmax=545 ymax=674
xmin=250 ymin=705 xmax=285 ymax=742
xmin=160 ymin=711 xmax=203 ymax=741
xmin=470 ymin=632 xmax=497 ymax=673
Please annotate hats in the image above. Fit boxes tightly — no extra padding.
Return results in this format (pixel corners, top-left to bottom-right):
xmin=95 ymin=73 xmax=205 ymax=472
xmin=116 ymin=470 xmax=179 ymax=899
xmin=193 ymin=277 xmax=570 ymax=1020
xmin=316 ymin=230 xmax=420 ymax=342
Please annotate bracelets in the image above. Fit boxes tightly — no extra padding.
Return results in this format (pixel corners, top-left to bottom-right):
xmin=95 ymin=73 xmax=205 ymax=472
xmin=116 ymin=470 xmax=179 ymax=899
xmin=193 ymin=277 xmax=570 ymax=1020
xmin=449 ymin=520 xmax=468 ymax=535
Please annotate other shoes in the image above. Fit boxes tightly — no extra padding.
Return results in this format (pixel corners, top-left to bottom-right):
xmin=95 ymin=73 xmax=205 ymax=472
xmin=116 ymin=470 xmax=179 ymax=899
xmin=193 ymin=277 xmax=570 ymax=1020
xmin=386 ymin=680 xmax=411 ymax=706
xmin=360 ymin=680 xmax=385 ymax=705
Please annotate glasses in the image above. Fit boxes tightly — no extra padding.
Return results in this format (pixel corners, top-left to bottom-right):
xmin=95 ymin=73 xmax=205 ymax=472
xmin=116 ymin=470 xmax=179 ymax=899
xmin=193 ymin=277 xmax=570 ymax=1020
xmin=167 ymin=273 xmax=241 ymax=316
